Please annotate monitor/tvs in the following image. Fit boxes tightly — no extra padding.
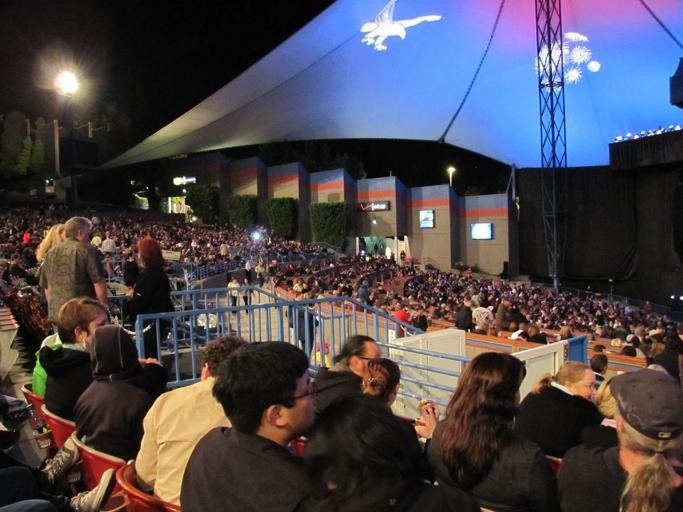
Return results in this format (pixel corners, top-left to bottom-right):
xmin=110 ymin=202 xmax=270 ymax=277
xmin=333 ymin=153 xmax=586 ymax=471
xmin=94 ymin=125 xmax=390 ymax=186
xmin=420 ymin=209 xmax=435 ymax=228
xmin=470 ymin=223 xmax=493 ymax=240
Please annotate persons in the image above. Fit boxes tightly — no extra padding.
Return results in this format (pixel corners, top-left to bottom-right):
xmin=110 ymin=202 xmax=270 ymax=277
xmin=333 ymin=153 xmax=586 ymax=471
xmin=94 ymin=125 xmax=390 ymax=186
xmin=360 ymin=356 xmax=441 ymax=457
xmin=512 ymin=360 xmax=620 ymax=461
xmin=1 ymin=193 xmax=683 ymax=427
xmin=70 ymin=323 xmax=167 ymax=462
xmin=422 ymin=349 xmax=557 ymax=512
xmin=1 ymin=398 xmax=116 ymax=511
xmin=288 ymin=389 xmax=482 ymax=511
xmin=176 ymin=340 xmax=318 ymax=511
xmin=550 ymin=367 xmax=682 ymax=511
xmin=131 ymin=333 xmax=249 ymax=509
xmin=311 ymin=334 xmax=380 ymax=418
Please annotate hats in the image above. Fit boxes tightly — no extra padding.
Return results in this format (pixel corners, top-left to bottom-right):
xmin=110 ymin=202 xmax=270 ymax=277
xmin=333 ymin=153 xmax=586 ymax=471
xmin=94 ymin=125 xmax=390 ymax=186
xmin=609 ymin=368 xmax=681 ymax=439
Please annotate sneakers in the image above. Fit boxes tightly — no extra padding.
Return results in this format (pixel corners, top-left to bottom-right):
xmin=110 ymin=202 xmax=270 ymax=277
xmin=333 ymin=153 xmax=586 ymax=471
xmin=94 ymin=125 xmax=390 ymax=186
xmin=70 ymin=468 xmax=117 ymax=512
xmin=42 ymin=438 xmax=81 ymax=485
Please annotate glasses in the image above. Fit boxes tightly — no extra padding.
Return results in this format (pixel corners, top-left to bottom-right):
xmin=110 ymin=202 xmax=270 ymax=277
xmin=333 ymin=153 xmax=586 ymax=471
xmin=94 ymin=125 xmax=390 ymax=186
xmin=295 ymin=381 xmax=320 ymax=399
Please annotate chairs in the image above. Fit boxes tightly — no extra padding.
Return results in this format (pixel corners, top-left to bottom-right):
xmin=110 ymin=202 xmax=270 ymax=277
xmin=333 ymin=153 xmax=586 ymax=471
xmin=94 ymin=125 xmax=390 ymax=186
xmin=70 ymin=430 xmax=127 ymax=497
xmin=115 ymin=458 xmax=183 ymax=512
xmin=21 ymin=383 xmax=47 ymax=431
xmin=40 ymin=403 xmax=76 ymax=450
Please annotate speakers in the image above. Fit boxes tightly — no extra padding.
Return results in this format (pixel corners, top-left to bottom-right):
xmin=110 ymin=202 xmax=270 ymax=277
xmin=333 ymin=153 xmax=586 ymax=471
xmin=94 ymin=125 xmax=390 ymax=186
xmin=63 ymin=138 xmax=98 ymax=170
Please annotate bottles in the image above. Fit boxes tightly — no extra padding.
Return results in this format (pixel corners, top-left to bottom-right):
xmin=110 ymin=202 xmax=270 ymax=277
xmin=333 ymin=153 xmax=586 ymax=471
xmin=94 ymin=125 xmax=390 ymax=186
xmin=25 ymin=403 xmax=52 ymax=449
